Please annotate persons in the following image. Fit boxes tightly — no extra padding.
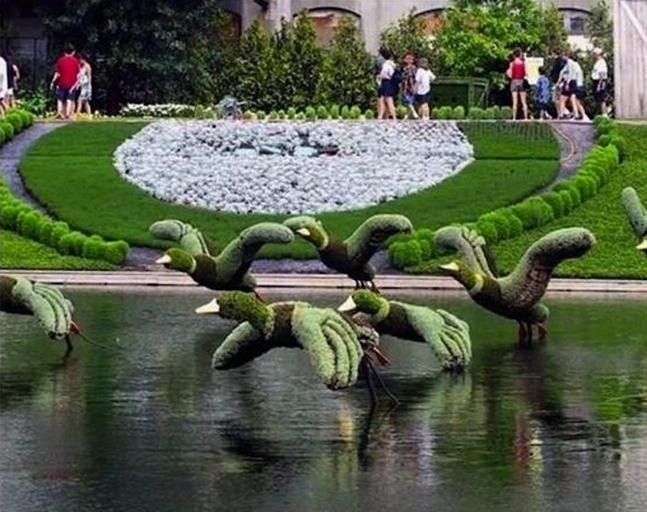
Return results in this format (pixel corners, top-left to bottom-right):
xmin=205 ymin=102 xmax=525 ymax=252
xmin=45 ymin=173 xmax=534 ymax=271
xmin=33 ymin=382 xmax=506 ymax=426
xmin=570 ymin=54 xmax=589 ymax=122
xmin=558 ymin=52 xmax=579 ymax=119
xmin=548 ymin=47 xmax=567 ymax=120
xmin=48 ymin=44 xmax=79 ymax=118
xmin=399 ymin=50 xmax=420 ymax=121
xmin=2 ymin=50 xmax=20 ymax=110
xmin=533 ymin=65 xmax=551 ymax=120
xmin=377 ymin=45 xmax=397 ymax=120
xmin=506 ymin=48 xmax=528 ymax=121
xmin=590 ymin=47 xmax=613 ymax=120
xmin=0 ymin=48 xmax=10 ymax=116
xmin=68 ymin=52 xmax=92 ymax=117
xmin=412 ymin=58 xmax=437 ymax=121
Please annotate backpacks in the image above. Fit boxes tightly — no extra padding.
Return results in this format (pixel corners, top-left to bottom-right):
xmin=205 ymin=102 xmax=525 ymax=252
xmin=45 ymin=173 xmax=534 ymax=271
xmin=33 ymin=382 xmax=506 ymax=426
xmin=387 ymin=62 xmax=403 ymax=85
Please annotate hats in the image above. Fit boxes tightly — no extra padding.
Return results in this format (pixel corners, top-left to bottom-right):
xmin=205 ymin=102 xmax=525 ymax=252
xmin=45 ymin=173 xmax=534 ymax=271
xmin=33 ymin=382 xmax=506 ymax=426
xmin=417 ymin=58 xmax=428 ymax=69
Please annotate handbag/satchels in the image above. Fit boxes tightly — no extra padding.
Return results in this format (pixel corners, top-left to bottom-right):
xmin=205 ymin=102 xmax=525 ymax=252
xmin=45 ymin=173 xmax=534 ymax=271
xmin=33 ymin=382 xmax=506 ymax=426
xmin=523 ymin=79 xmax=531 ymax=89
